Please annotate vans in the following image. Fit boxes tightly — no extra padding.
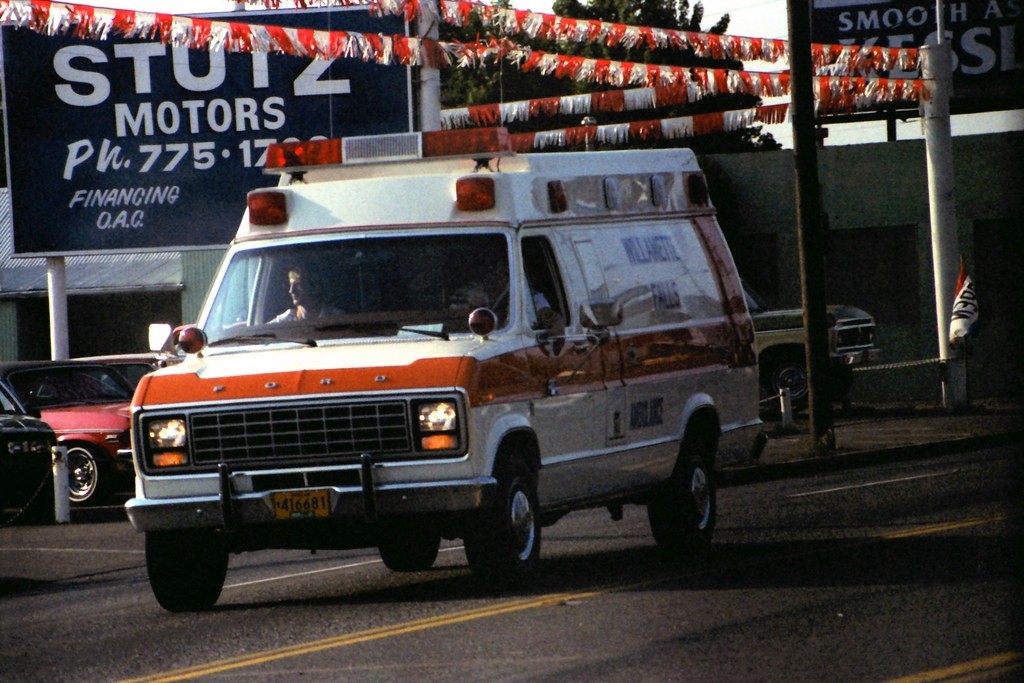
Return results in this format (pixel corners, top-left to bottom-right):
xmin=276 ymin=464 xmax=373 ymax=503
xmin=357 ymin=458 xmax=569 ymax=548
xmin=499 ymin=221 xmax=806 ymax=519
xmin=122 ymin=127 xmax=771 ymax=616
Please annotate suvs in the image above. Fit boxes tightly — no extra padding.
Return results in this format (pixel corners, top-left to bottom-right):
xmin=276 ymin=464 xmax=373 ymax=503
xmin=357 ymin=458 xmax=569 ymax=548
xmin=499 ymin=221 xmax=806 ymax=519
xmin=739 ymin=288 xmax=882 ymax=424
xmin=0 ymin=348 xmax=190 ymax=529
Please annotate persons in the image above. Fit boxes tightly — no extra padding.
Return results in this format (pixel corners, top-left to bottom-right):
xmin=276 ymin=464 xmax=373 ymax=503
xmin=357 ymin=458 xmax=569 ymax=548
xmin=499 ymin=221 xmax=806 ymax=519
xmin=263 ymin=259 xmax=347 ymax=326
xmin=447 ymin=243 xmax=565 ymax=336
